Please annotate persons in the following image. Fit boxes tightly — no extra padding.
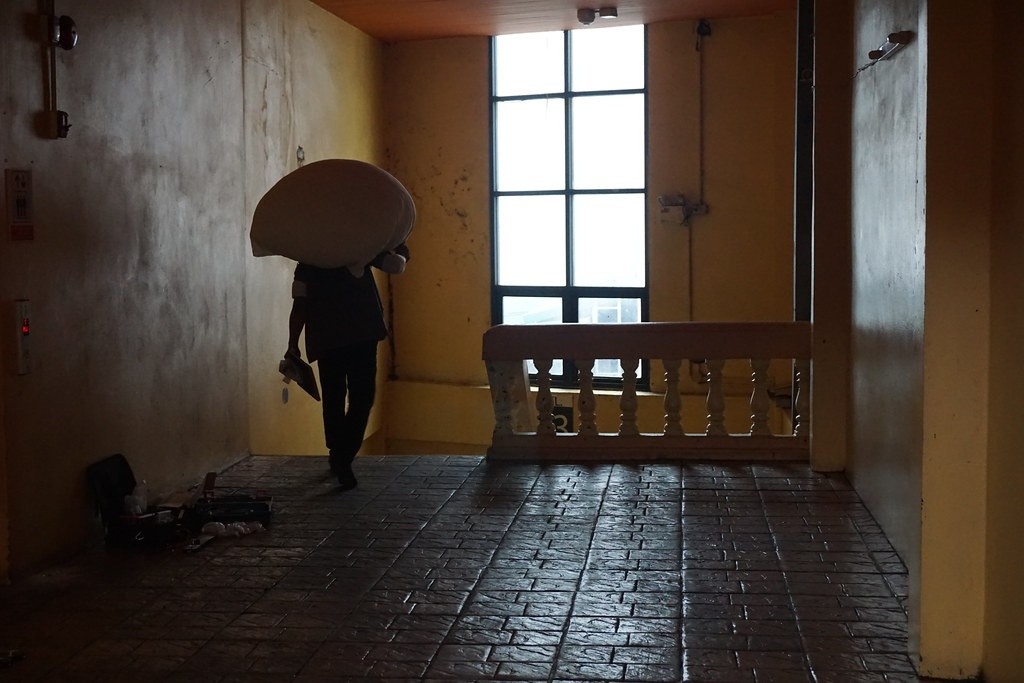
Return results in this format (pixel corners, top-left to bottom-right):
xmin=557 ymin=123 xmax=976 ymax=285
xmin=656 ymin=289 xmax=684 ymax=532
xmin=283 ymin=249 xmax=407 ymax=488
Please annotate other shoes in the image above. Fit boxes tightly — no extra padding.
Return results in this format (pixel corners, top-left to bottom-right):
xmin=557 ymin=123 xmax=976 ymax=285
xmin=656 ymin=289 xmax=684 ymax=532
xmin=327 ymin=449 xmax=355 ymax=485
